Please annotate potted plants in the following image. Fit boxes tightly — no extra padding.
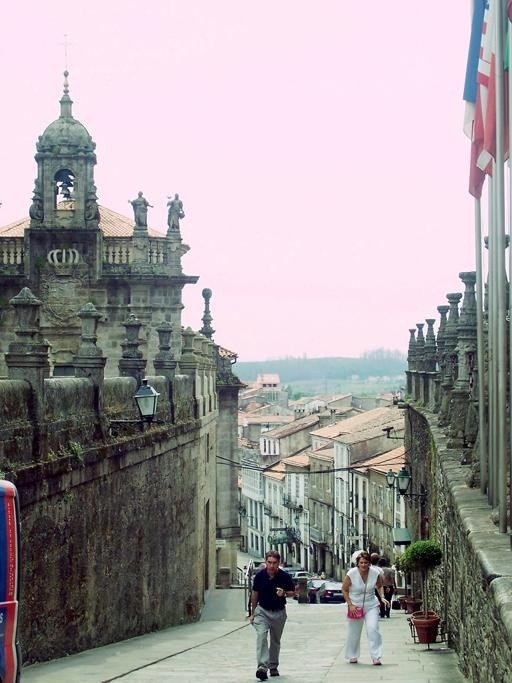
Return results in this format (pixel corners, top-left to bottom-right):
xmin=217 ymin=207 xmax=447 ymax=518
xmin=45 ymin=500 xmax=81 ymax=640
xmin=394 ymin=553 xmax=423 ymax=614
xmin=405 ymin=544 xmax=437 ymax=616
xmin=410 ymin=539 xmax=443 ymax=643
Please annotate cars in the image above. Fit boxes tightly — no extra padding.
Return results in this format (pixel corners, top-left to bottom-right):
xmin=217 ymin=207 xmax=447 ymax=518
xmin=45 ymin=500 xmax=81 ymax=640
xmin=286 ymin=569 xmax=346 ymax=603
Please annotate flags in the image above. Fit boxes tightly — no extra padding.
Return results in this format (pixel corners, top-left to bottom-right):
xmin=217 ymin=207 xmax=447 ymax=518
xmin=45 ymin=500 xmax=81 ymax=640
xmin=460 ymin=0 xmax=512 ymax=201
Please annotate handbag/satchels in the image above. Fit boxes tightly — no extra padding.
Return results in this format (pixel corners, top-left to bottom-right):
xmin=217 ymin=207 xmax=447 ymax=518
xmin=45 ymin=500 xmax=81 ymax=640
xmin=348 ymin=607 xmax=364 ymax=619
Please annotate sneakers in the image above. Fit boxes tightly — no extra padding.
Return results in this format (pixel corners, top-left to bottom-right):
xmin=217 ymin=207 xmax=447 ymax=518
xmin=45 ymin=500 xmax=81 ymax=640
xmin=270 ymin=669 xmax=279 ymax=676
xmin=256 ymin=669 xmax=268 ymax=681
xmin=350 ymin=658 xmax=357 ymax=663
xmin=373 ymin=659 xmax=381 ymax=665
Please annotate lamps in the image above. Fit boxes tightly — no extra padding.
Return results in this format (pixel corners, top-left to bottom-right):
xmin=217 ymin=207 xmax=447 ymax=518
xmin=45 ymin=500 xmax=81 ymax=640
xmin=383 ymin=467 xmax=396 ymax=490
xmin=396 ymin=465 xmax=426 ymax=497
xmin=109 ymin=379 xmax=164 ymax=425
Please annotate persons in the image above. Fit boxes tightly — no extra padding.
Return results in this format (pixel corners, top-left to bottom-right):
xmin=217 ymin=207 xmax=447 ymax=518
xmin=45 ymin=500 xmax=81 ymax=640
xmin=341 ymin=552 xmax=391 ymax=665
xmin=249 ymin=550 xmax=295 ymax=680
xmin=370 ymin=552 xmax=397 ymax=618
